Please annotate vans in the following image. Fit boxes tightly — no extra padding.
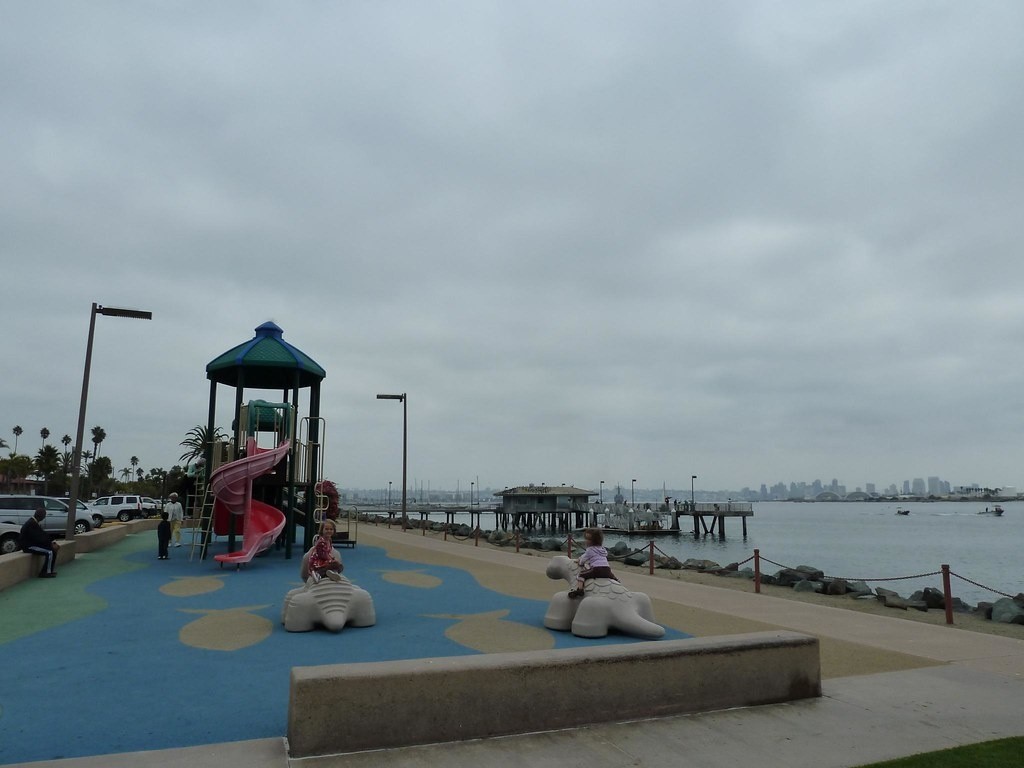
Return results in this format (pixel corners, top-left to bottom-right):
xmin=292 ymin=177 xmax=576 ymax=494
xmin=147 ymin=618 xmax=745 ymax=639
xmin=1 ymin=494 xmax=94 ymax=537
xmin=88 ymin=494 xmax=142 ymax=521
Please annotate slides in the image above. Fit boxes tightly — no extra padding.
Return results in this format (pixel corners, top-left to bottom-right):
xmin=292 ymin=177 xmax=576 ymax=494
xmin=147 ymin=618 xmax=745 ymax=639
xmin=209 ymin=436 xmax=288 ymax=564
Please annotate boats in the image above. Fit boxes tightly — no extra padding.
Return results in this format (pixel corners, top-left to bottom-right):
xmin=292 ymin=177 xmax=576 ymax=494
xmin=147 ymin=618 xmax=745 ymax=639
xmin=897 ymin=510 xmax=911 ymax=516
xmin=975 ymin=504 xmax=1005 ymax=517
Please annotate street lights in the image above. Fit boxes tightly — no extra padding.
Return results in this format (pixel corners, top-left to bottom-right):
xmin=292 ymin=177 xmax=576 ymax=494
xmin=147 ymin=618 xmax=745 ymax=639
xmin=469 ymin=481 xmax=475 ymax=504
xmin=691 ymin=474 xmax=698 ymax=510
xmin=64 ymin=299 xmax=154 ymax=547
xmin=631 ymin=478 xmax=637 ymax=509
xmin=377 ymin=392 xmax=410 ymax=532
xmin=600 ymin=480 xmax=605 ymax=502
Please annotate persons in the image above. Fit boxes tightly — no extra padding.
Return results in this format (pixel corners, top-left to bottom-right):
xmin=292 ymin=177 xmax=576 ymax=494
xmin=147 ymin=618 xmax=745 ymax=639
xmin=157 ymin=512 xmax=172 ymax=559
xmin=309 ymin=518 xmax=344 ymax=575
xmin=568 ymin=528 xmax=621 ymax=598
xmin=20 ymin=508 xmax=59 ymax=578
xmin=164 ymin=492 xmax=183 ymax=547
xmin=592 ymin=497 xmax=733 ymax=511
xmin=985 ymin=504 xmax=1002 ymax=512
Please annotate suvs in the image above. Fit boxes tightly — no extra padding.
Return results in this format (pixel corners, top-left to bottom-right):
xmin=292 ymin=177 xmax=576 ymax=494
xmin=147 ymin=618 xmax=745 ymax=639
xmin=139 ymin=497 xmax=157 ymax=519
xmin=49 ymin=497 xmax=104 ymax=530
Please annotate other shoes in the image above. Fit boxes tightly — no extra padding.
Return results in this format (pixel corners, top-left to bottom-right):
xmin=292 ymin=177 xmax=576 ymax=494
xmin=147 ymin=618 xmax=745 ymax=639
xmin=39 ymin=572 xmax=58 ymax=578
xmin=326 ymin=570 xmax=341 ymax=581
xmin=311 ymin=571 xmax=320 ymax=583
xmin=168 ymin=544 xmax=172 ymax=547
xmin=158 ymin=555 xmax=166 ymax=559
xmin=174 ymin=543 xmax=181 ymax=547
xmin=568 ymin=587 xmax=584 ymax=598
